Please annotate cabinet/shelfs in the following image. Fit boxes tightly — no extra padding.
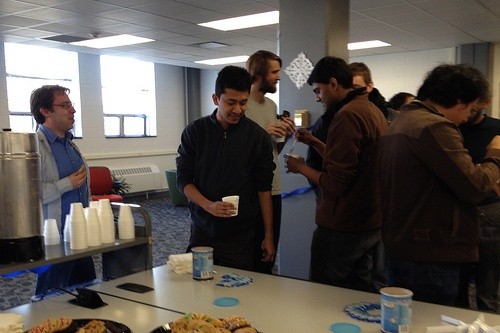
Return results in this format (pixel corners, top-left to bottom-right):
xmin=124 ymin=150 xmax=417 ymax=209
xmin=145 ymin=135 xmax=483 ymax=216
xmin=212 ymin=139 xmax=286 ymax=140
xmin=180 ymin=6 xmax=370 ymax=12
xmin=0 ymin=201 xmax=152 ymax=280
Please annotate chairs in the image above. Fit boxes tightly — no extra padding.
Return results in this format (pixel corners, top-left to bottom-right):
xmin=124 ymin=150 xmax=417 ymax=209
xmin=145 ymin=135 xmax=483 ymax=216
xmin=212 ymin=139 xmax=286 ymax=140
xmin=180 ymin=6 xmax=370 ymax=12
xmin=89 ymin=165 xmax=123 ymax=203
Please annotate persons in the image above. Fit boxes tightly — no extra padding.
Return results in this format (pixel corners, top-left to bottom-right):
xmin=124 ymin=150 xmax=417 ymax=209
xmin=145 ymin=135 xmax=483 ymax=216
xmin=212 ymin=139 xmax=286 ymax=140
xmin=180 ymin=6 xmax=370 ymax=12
xmin=280 ymin=56 xmax=500 ymax=316
xmin=31 ymin=86 xmax=97 ymax=286
xmin=176 ymin=66 xmax=277 ymax=275
xmin=245 ymin=50 xmax=297 ymax=275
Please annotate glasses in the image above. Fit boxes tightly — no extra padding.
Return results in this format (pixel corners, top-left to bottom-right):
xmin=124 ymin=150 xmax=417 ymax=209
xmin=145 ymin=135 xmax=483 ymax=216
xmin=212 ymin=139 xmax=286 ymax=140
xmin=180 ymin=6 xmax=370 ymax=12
xmin=50 ymin=102 xmax=74 ymax=109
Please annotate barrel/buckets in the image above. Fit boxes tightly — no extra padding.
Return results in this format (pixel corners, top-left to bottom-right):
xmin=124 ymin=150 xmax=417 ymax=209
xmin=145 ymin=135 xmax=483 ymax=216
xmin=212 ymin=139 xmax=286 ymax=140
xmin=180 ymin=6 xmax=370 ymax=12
xmin=0 ymin=129 xmax=45 ymax=262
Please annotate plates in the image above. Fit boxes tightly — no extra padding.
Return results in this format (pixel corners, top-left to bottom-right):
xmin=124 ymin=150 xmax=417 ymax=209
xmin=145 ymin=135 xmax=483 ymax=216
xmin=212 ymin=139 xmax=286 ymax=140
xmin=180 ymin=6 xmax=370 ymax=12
xmin=23 ymin=318 xmax=133 ymax=333
xmin=150 ymin=319 xmax=260 ymax=333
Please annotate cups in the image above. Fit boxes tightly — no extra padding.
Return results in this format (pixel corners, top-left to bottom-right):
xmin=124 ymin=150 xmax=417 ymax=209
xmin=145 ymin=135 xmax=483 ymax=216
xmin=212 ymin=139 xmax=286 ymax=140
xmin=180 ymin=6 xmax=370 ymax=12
xmin=287 ymin=153 xmax=299 ymax=158
xmin=222 ymin=195 xmax=239 ymax=216
xmin=191 ymin=247 xmax=213 ymax=281
xmin=45 ymin=199 xmax=135 ymax=249
xmin=275 ymin=136 xmax=285 ymax=142
xmin=380 ymin=287 xmax=413 ymax=333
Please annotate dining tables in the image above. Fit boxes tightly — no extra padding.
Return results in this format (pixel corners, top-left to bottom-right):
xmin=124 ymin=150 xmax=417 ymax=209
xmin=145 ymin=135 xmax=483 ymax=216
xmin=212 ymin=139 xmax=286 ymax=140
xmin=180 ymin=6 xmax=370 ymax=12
xmin=0 ymin=264 xmax=500 ymax=333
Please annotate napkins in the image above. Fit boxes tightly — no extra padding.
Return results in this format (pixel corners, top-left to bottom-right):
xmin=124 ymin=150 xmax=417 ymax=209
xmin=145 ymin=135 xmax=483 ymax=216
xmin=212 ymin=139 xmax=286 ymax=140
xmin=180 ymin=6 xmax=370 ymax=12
xmin=167 ymin=253 xmax=192 ymax=275
